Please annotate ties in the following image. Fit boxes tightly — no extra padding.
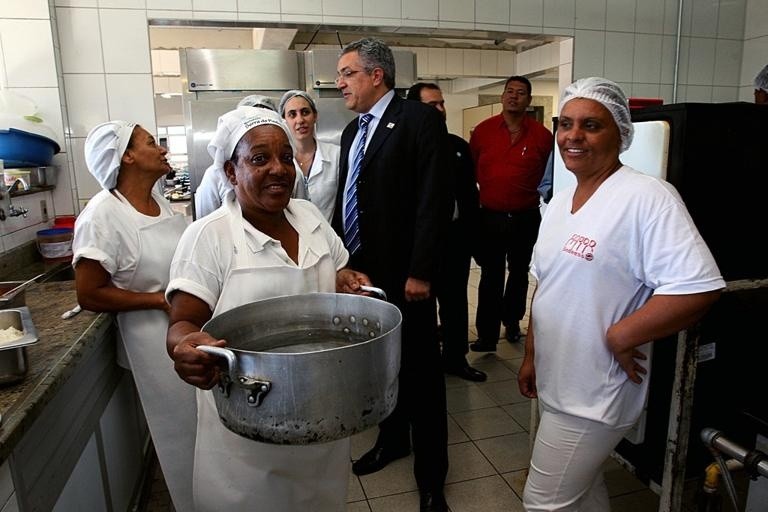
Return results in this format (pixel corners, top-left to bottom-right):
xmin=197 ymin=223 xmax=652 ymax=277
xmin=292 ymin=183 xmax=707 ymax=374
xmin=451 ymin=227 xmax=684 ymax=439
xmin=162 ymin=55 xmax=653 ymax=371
xmin=344 ymin=113 xmax=374 ymax=255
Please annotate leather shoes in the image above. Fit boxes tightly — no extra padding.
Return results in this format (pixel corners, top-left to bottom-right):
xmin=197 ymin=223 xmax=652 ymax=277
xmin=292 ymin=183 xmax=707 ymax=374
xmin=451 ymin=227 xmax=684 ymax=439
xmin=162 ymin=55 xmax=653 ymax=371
xmin=455 ymin=364 xmax=487 ymax=382
xmin=505 ymin=325 xmax=521 ymax=343
xmin=352 ymin=442 xmax=409 ymax=476
xmin=419 ymin=491 xmax=448 ymax=512
xmin=471 ymin=340 xmax=496 ymax=352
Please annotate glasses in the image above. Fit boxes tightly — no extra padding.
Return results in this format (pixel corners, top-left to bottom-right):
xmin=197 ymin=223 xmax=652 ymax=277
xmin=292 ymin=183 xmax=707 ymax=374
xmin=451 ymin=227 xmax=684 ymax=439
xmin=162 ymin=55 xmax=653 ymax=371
xmin=334 ymin=70 xmax=364 ymax=85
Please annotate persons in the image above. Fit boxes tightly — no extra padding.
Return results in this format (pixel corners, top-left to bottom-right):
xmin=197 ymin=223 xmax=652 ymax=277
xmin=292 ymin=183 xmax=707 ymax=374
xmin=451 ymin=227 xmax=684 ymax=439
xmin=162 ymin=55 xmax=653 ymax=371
xmin=516 ymin=77 xmax=728 ymax=511
xmin=753 ymin=64 xmax=768 ymax=105
xmin=330 ymin=37 xmax=452 ymax=512
xmin=279 ymin=89 xmax=341 ymax=228
xmin=407 ymin=81 xmax=488 ymax=383
xmin=468 ymin=76 xmax=555 ymax=352
xmin=194 ymin=94 xmax=278 ymax=220
xmin=166 ymin=106 xmax=374 ymax=511
xmin=70 ymin=121 xmax=199 ymax=511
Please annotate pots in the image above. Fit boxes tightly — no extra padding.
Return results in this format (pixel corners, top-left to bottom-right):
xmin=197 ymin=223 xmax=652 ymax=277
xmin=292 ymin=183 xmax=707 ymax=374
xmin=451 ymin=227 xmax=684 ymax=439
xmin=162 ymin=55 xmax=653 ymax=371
xmin=194 ymin=285 xmax=403 ymax=446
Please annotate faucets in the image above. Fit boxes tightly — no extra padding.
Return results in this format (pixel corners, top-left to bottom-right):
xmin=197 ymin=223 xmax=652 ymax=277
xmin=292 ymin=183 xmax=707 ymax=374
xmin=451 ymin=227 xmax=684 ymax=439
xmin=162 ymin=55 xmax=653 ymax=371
xmin=5 ymin=177 xmax=30 ymax=193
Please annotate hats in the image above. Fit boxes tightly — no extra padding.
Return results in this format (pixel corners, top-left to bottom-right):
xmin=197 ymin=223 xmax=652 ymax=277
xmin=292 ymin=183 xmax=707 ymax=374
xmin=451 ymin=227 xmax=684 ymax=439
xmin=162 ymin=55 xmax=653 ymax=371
xmin=84 ymin=120 xmax=137 ymax=192
xmin=207 ymin=106 xmax=298 ymax=190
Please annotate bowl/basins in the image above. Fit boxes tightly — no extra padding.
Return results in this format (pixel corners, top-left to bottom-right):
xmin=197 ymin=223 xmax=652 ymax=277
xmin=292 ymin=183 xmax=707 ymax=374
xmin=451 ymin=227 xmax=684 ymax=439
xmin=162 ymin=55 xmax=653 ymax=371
xmin=0 ymin=127 xmax=61 ymax=168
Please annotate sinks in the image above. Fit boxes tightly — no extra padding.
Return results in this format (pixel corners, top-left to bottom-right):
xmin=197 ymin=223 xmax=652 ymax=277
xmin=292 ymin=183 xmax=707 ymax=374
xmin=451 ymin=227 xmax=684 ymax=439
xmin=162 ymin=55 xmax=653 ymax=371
xmin=35 ymin=260 xmax=74 ymax=282
